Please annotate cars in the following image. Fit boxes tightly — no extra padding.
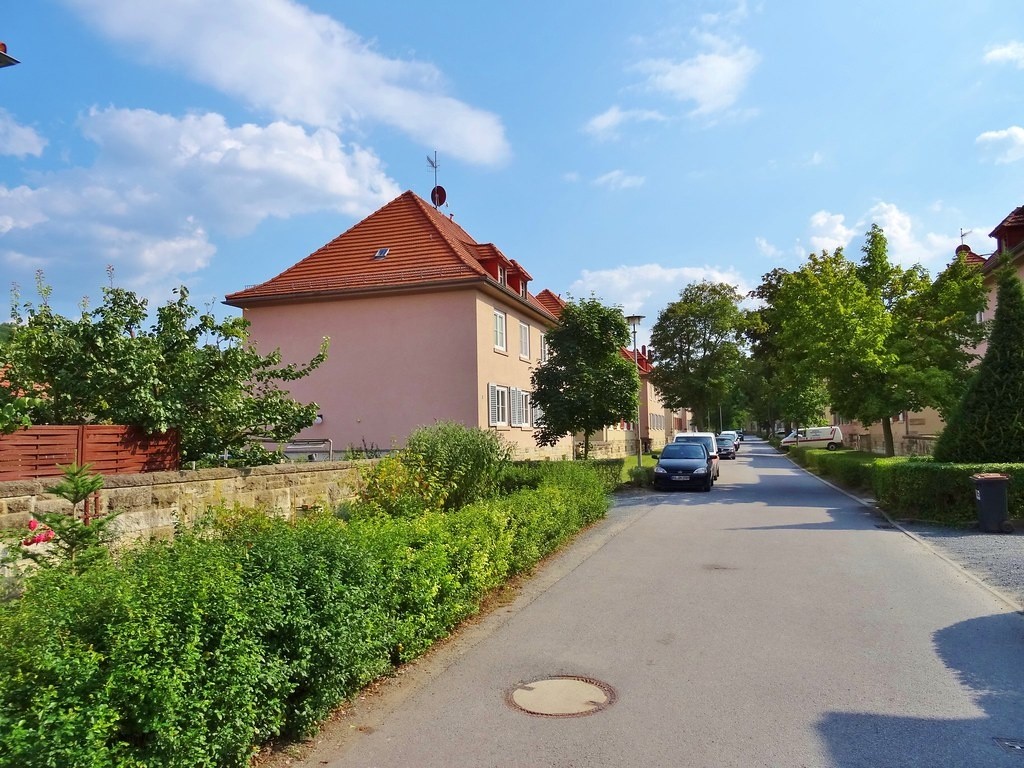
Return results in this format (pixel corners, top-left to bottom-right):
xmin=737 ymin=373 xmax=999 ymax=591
xmin=715 ymin=437 xmax=735 ymax=460
xmin=768 ymin=432 xmax=787 ymax=441
xmin=720 ymin=431 xmax=743 ymax=451
xmin=652 ymin=442 xmax=717 ymax=492
xmin=672 ymin=432 xmax=719 ymax=480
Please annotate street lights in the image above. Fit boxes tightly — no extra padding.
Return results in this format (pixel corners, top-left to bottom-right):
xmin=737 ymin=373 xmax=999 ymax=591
xmin=624 ymin=313 xmax=646 ymax=467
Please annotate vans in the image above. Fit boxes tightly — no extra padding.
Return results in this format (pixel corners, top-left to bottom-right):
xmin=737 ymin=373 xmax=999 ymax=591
xmin=779 ymin=427 xmax=844 ymax=451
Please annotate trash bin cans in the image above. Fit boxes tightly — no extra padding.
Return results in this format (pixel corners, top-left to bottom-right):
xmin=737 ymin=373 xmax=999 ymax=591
xmin=969 ymin=472 xmax=1015 ymax=534
